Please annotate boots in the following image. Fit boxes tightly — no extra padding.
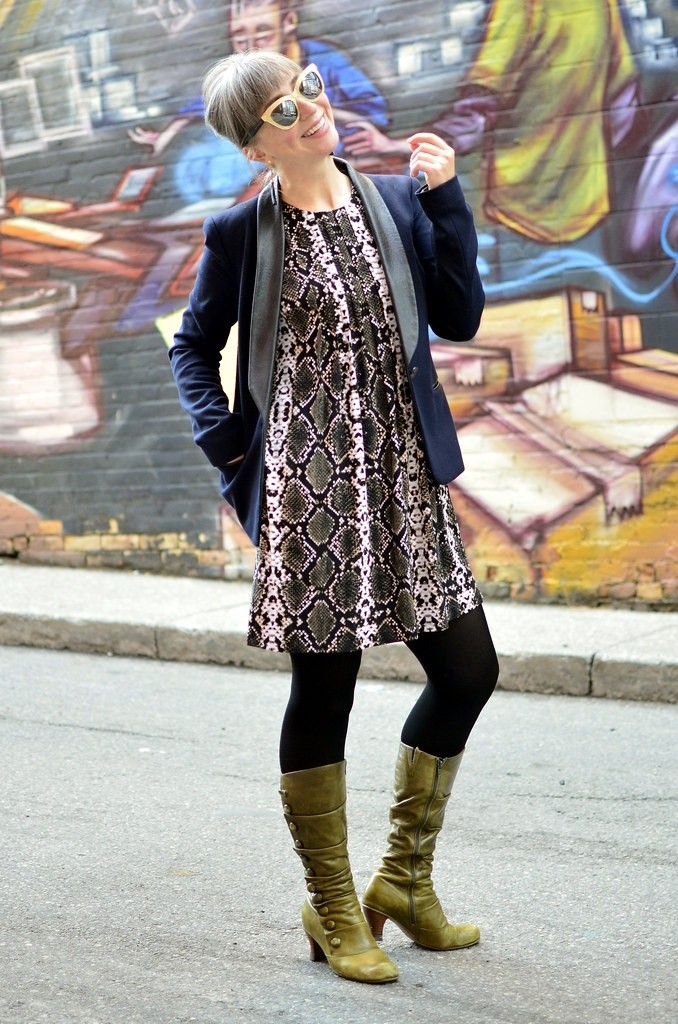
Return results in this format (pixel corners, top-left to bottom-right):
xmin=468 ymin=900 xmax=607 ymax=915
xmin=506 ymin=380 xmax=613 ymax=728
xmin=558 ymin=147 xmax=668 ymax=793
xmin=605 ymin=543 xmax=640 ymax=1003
xmin=361 ymin=740 xmax=481 ymax=952
xmin=277 ymin=759 xmax=399 ymax=983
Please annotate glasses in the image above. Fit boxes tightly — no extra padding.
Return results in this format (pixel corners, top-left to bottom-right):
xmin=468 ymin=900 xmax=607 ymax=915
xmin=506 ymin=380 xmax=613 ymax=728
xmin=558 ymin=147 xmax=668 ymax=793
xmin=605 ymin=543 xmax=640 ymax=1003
xmin=239 ymin=63 xmax=325 ymax=148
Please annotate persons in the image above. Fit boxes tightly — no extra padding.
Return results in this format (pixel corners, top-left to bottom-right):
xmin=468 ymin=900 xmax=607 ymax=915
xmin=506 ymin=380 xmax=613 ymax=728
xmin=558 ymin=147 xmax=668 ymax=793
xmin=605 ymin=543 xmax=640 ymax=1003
xmin=168 ymin=50 xmax=500 ymax=985
